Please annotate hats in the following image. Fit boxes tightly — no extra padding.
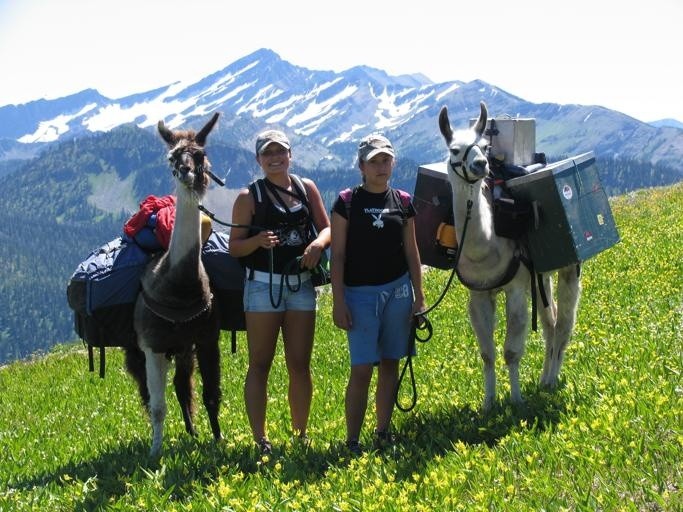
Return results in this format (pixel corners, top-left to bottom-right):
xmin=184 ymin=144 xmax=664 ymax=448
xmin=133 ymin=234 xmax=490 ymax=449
xmin=358 ymin=134 xmax=395 ymax=162
xmin=256 ymin=130 xmax=291 ymax=155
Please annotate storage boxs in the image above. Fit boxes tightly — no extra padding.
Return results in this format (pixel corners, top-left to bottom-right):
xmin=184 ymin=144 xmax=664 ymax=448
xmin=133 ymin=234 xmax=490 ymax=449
xmin=506 ymin=150 xmax=621 ymax=275
xmin=412 ymin=161 xmax=458 ymax=270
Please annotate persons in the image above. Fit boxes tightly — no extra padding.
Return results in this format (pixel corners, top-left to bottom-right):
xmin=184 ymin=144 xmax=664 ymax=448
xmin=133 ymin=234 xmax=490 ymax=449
xmin=329 ymin=133 xmax=426 ymax=454
xmin=229 ymin=129 xmax=331 ymax=450
